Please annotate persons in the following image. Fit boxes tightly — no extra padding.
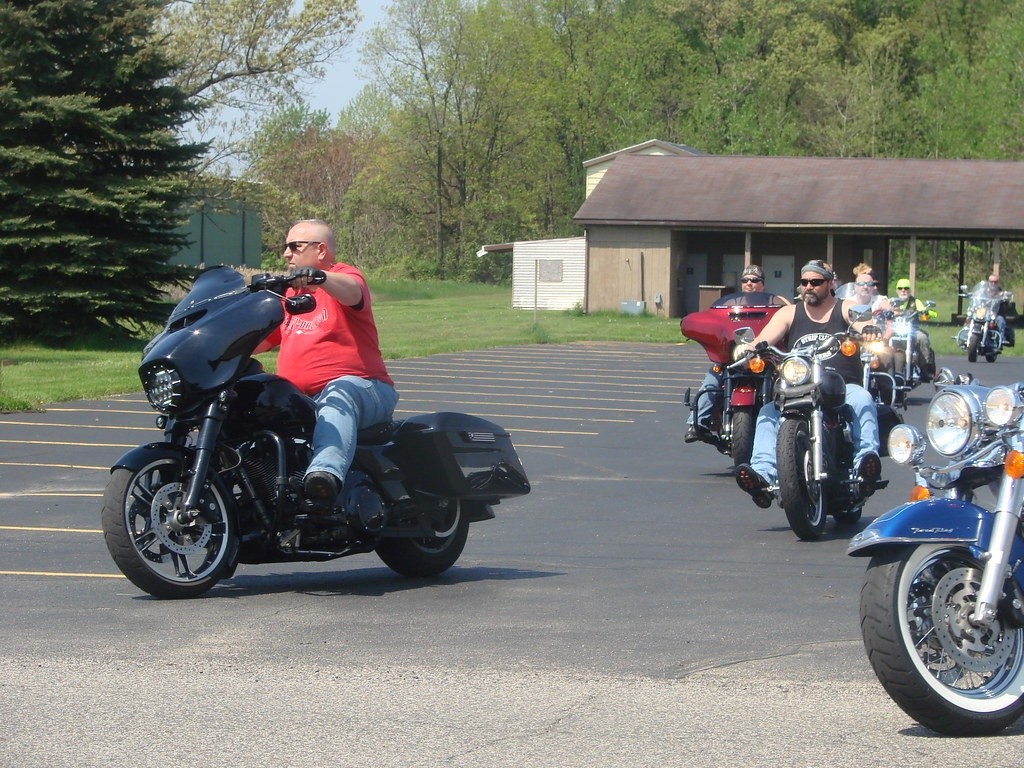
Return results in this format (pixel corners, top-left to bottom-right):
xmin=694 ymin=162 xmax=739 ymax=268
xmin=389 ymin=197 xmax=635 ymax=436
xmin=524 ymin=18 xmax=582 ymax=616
xmin=680 ymin=264 xmax=793 ymax=442
xmin=251 ymin=219 xmax=400 ymax=516
xmin=734 ymin=260 xmax=882 ymax=508
xmin=852 ymin=263 xmax=907 ymax=400
xmin=959 ymin=275 xmax=1018 ymax=350
xmin=889 ymin=279 xmax=938 ymax=380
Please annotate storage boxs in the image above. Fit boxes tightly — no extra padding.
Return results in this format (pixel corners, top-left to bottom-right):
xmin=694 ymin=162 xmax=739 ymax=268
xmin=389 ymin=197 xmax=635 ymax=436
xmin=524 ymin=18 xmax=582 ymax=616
xmin=392 ymin=409 xmax=529 ymax=502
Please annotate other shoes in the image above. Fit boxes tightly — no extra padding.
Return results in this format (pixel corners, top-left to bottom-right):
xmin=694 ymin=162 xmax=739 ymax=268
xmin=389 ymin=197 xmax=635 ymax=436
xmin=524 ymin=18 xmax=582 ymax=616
xmin=735 ymin=463 xmax=775 ymax=509
xmin=304 ymin=472 xmax=341 ymax=526
xmin=859 ymin=452 xmax=881 ymax=497
xmin=685 ymin=426 xmax=711 ymax=444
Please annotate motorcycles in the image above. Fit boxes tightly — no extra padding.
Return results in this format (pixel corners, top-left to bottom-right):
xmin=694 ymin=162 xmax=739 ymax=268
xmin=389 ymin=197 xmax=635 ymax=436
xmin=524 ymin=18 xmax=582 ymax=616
xmin=835 ymin=282 xmax=936 ymax=411
xmin=680 ymin=294 xmax=786 ymax=469
xmin=951 ymin=280 xmax=1012 ymax=364
xmin=726 ymin=304 xmax=905 ymax=542
xmin=102 ymin=265 xmax=531 ymax=600
xmin=844 ymin=366 xmax=1024 ymax=737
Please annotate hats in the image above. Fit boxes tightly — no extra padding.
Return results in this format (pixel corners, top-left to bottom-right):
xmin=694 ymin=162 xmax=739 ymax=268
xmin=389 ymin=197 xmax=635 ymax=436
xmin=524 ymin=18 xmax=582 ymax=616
xmin=742 ymin=265 xmax=765 ymax=280
xmin=896 ymin=279 xmax=911 ymax=287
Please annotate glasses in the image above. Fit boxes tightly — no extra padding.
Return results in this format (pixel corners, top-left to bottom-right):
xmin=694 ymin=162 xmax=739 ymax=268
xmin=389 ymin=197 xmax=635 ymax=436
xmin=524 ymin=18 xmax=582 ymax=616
xmin=284 ymin=241 xmax=321 ymax=251
xmin=854 ymin=281 xmax=874 ymax=286
xmin=898 ymin=287 xmax=909 ymax=290
xmin=741 ymin=277 xmax=764 ymax=283
xmin=800 ymin=279 xmax=827 ymax=286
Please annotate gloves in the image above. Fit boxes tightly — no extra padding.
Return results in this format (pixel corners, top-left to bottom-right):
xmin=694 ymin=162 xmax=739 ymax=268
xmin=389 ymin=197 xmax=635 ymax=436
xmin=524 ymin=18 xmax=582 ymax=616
xmin=928 ymin=310 xmax=938 ymax=319
